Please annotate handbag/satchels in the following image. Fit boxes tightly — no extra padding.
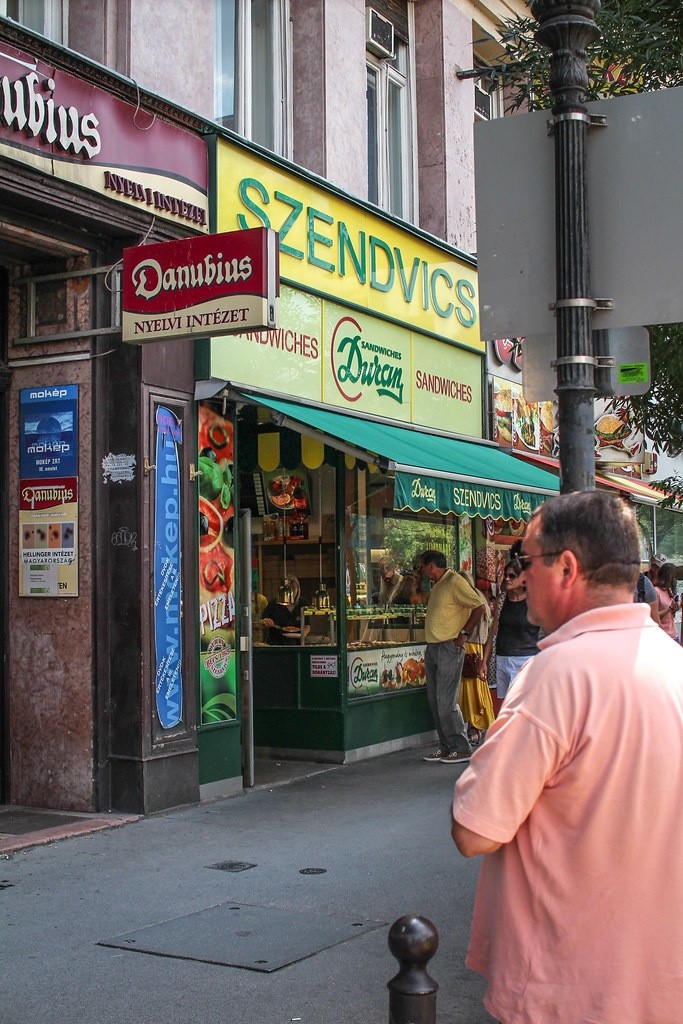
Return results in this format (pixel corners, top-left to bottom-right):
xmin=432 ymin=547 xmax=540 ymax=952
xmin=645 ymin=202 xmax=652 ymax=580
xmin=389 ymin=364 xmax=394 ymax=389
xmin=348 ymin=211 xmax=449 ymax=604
xmin=462 ymin=653 xmax=482 ymax=678
xmin=489 ymin=633 xmax=498 ymax=685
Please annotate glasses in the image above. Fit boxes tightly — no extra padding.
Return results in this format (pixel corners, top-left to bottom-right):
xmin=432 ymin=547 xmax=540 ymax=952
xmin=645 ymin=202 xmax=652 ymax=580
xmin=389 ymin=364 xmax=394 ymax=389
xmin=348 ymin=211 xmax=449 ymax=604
xmin=504 ymin=572 xmax=519 ymax=579
xmin=515 ymin=550 xmax=565 ymax=570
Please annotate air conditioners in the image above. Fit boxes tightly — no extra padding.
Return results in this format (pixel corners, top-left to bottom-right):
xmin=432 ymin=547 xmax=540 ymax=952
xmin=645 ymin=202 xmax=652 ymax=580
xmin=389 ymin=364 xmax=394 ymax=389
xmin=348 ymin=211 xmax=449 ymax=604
xmin=366 ymin=6 xmax=394 ymax=60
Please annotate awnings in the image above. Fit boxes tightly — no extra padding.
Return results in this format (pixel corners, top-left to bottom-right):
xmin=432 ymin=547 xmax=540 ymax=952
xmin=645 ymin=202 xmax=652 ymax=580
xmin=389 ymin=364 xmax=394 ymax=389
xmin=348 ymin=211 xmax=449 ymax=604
xmin=244 ymin=392 xmax=560 ymax=523
xmin=524 ymin=456 xmax=683 ymax=513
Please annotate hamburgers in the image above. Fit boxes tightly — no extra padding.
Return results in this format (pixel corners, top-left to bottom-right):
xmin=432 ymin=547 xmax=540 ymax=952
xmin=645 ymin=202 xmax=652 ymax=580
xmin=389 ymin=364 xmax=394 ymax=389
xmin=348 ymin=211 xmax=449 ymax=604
xmin=494 ymin=389 xmax=512 ymax=441
xmin=594 ymin=414 xmax=632 ymax=449
xmin=539 ymin=401 xmax=553 ymax=452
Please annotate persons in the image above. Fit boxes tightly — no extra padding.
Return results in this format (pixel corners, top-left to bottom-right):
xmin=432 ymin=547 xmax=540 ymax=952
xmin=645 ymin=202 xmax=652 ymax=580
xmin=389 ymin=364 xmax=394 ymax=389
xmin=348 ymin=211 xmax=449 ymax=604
xmin=251 ymin=575 xmax=310 ymax=645
xmin=451 ymin=491 xmax=683 ymax=1024
xmin=420 ymin=549 xmax=683 ymax=763
xmin=362 ymin=554 xmax=431 ymax=605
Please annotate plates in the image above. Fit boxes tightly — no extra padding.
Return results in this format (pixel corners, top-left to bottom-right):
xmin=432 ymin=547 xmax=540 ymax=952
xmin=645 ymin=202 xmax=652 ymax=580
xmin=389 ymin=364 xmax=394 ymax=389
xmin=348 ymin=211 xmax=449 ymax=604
xmin=283 ymin=633 xmax=306 ymax=639
xmin=267 ymin=485 xmax=296 ymax=510
xmin=514 ymin=415 xmax=539 ymax=451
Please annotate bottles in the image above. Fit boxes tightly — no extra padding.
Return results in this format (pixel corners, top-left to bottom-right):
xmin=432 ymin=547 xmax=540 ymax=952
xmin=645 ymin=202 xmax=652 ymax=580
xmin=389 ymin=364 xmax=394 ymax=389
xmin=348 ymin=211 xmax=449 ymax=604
xmin=293 ymin=476 xmax=307 ymax=514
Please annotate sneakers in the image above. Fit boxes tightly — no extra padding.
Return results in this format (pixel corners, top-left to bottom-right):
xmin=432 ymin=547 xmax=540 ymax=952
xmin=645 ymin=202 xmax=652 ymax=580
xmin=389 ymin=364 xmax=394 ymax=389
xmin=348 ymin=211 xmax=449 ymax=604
xmin=423 ymin=748 xmax=450 ymax=761
xmin=440 ymin=751 xmax=474 ymax=763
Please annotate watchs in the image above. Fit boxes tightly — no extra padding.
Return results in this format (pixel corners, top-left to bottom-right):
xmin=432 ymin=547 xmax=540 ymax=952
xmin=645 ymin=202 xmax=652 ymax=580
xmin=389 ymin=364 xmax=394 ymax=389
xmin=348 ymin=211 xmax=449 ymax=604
xmin=460 ymin=630 xmax=469 ymax=636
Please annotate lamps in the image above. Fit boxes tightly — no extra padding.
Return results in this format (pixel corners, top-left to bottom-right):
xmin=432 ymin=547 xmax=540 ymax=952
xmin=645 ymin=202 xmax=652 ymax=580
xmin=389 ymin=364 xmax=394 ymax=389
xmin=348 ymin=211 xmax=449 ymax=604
xmin=312 ymin=466 xmax=330 ymax=611
xmin=278 ymin=468 xmax=295 ymax=604
